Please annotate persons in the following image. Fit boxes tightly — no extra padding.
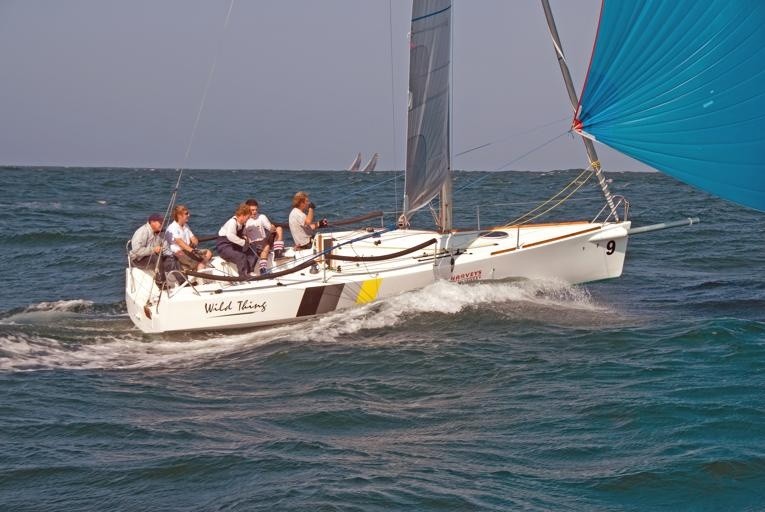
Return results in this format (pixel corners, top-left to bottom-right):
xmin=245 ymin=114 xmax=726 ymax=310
xmin=131 ymin=213 xmax=196 ymax=289
xmin=288 ymin=191 xmax=328 ymax=249
xmin=166 ymin=205 xmax=213 ymax=271
xmin=245 ymin=199 xmax=286 ymax=275
xmin=216 ymin=203 xmax=257 ymax=278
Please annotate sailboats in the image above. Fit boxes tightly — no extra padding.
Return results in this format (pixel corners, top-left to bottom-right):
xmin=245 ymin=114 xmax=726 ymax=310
xmin=121 ymin=0 xmax=760 ymax=340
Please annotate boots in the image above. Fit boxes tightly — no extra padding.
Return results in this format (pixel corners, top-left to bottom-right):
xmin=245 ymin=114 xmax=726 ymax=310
xmin=260 ymin=268 xmax=271 ymax=275
xmin=274 ymin=250 xmax=285 ymax=260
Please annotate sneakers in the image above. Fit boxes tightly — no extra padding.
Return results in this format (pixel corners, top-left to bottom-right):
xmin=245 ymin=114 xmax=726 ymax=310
xmin=248 ymin=272 xmax=256 ymax=277
xmin=180 ymin=279 xmax=197 ymax=287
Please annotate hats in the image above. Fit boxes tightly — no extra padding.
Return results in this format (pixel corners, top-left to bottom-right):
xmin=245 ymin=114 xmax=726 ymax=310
xmin=149 ymin=214 xmax=164 ymax=223
